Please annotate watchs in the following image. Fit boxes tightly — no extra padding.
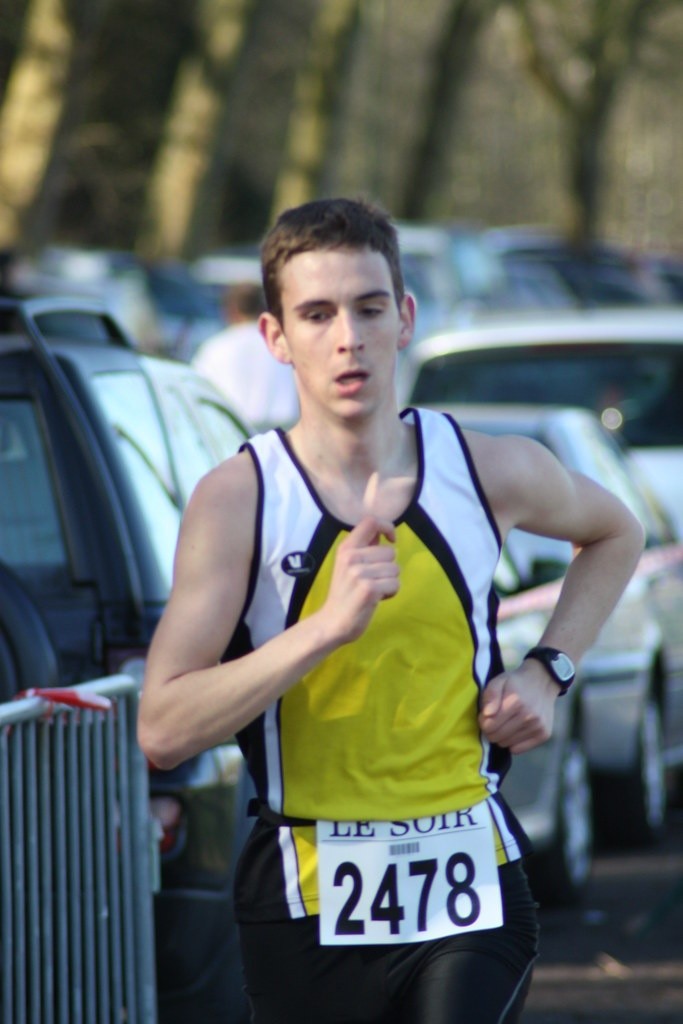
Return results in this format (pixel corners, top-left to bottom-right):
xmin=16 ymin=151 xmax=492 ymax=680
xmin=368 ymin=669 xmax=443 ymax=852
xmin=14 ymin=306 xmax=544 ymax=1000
xmin=524 ymin=647 xmax=576 ymax=696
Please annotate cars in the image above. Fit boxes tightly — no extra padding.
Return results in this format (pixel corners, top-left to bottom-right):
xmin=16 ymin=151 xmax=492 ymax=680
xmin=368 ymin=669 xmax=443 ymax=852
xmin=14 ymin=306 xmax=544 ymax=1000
xmin=1 ymin=211 xmax=682 ymax=906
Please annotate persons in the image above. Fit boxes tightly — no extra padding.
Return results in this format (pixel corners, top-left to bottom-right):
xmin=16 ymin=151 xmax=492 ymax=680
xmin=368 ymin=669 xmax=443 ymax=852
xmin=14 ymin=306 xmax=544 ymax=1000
xmin=135 ymin=200 xmax=645 ymax=1024
xmin=187 ymin=285 xmax=301 ymax=434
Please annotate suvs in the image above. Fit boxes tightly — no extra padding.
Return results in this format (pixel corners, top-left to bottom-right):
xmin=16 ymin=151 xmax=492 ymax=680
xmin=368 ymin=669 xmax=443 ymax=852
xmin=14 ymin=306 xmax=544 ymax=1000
xmin=1 ymin=287 xmax=265 ymax=1024
